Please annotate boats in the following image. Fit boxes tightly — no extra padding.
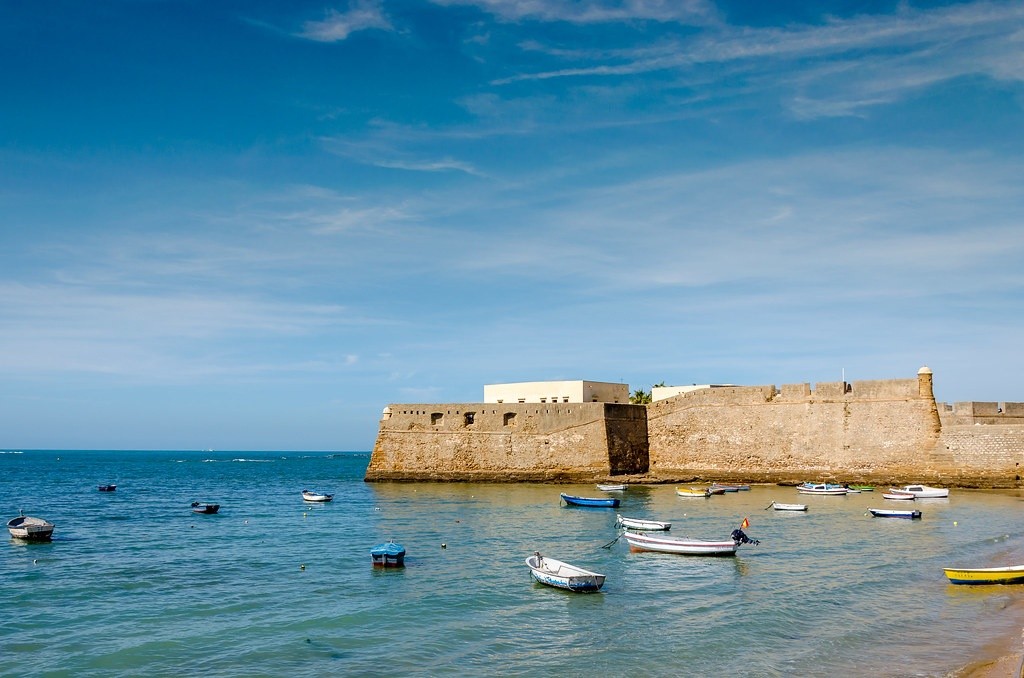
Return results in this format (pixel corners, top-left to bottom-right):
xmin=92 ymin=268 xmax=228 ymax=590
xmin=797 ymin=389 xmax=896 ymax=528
xmin=370 ymin=538 xmax=406 ymax=567
xmin=616 ymin=514 xmax=671 ymax=531
xmin=596 ymin=484 xmax=629 ymax=491
xmin=6 ymin=509 xmax=55 ymax=539
xmin=301 ymin=489 xmax=336 ymax=502
xmin=622 ymin=527 xmax=761 ymax=556
xmin=525 ymin=550 xmax=606 ymax=594
xmin=882 ymin=492 xmax=915 ymax=500
xmin=191 ymin=501 xmax=220 ymax=513
xmin=765 ymin=501 xmax=809 ymax=511
xmin=691 ymin=481 xmax=751 ymax=495
xmin=559 ymin=493 xmax=621 ymax=508
xmin=889 ymin=484 xmax=950 ymax=498
xmin=99 ymin=484 xmax=117 ymax=491
xmin=941 ymin=564 xmax=1024 ymax=585
xmin=795 ymin=481 xmax=876 ymax=495
xmin=867 ymin=507 xmax=922 ymax=518
xmin=675 ymin=486 xmax=713 ymax=497
